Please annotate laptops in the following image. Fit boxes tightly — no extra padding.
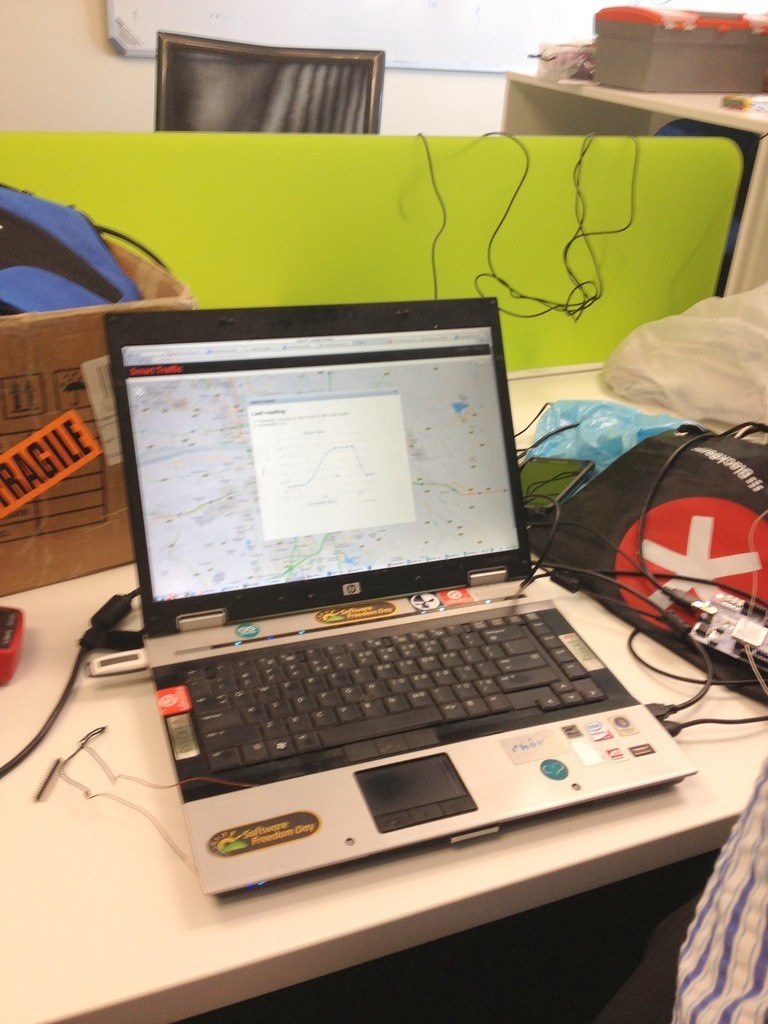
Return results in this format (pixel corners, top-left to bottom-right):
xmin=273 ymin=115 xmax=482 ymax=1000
xmin=101 ymin=298 xmax=697 ymax=894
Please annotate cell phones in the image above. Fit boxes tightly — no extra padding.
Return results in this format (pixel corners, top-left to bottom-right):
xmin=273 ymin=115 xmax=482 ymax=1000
xmin=519 ymin=457 xmax=595 ymax=515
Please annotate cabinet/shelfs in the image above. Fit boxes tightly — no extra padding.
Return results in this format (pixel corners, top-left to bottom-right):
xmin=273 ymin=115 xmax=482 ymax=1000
xmin=501 ymin=72 xmax=767 ymax=300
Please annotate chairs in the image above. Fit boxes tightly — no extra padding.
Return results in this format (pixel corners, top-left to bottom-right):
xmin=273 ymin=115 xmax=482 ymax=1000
xmin=153 ymin=29 xmax=386 ymax=135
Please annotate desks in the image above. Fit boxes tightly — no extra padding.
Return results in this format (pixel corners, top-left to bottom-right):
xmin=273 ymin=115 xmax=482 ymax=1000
xmin=0 ymin=359 xmax=767 ymax=1024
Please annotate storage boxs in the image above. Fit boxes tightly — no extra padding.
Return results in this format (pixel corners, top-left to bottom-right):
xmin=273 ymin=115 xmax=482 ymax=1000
xmin=0 ymin=241 xmax=192 ymax=601
xmin=591 ymin=6 xmax=768 ymax=92
